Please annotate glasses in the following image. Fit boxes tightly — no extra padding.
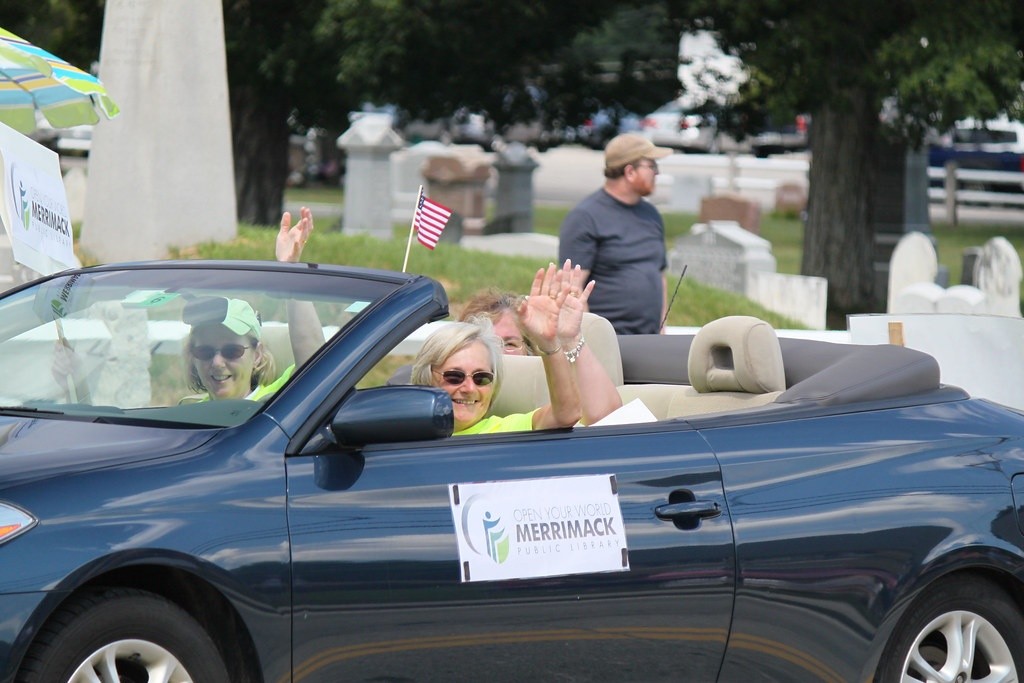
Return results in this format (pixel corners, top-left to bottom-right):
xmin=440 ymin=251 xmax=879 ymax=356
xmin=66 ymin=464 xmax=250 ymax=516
xmin=431 ymin=369 xmax=494 ymax=386
xmin=501 ymin=340 xmax=525 ymax=352
xmin=638 ymin=163 xmax=657 ymax=171
xmin=191 ymin=344 xmax=252 ymax=360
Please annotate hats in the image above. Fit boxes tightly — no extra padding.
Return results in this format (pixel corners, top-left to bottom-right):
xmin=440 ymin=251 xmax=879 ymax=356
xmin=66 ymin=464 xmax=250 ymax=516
xmin=182 ymin=297 xmax=261 ymax=342
xmin=605 ymin=134 xmax=673 ymax=168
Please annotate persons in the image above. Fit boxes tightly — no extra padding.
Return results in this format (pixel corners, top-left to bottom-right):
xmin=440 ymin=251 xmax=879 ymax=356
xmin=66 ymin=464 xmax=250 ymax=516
xmin=459 ymin=258 xmax=622 ymax=426
xmin=52 ymin=207 xmax=327 ymax=405
xmin=414 ymin=265 xmax=582 ymax=436
xmin=558 ymin=133 xmax=674 ymax=334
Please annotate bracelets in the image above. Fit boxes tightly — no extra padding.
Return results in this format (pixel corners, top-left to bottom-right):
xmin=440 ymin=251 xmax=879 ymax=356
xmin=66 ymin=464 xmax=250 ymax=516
xmin=563 ymin=335 xmax=585 ymax=364
xmin=537 ymin=345 xmax=560 ymax=355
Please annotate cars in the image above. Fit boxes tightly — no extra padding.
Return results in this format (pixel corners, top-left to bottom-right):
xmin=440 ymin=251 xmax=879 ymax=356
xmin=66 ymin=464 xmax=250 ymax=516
xmin=350 ymin=89 xmax=1024 ymax=190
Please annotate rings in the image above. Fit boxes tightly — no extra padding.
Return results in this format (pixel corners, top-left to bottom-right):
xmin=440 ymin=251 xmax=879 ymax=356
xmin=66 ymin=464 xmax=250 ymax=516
xmin=570 ymin=292 xmax=577 ymax=295
xmin=550 ymin=295 xmax=557 ymax=299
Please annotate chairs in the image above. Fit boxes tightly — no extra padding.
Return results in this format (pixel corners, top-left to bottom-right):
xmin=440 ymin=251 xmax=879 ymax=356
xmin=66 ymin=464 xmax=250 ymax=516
xmin=260 ymin=325 xmax=295 ymax=384
xmin=662 ymin=316 xmax=794 ymax=410
xmin=576 ymin=312 xmax=622 ymax=390
xmin=489 ymin=353 xmax=549 ymax=415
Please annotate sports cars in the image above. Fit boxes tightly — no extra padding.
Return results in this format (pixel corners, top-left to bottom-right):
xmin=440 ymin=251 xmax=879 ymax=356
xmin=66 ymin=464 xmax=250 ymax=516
xmin=0 ymin=260 xmax=1024 ymax=683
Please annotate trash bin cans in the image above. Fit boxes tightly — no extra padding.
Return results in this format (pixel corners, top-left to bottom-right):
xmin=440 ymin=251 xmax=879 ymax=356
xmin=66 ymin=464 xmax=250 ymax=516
xmin=423 ymin=153 xmax=540 ymax=240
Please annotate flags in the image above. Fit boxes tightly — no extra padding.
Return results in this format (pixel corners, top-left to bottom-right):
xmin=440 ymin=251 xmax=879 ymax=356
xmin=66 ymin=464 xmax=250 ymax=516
xmin=414 ymin=187 xmax=452 ymax=249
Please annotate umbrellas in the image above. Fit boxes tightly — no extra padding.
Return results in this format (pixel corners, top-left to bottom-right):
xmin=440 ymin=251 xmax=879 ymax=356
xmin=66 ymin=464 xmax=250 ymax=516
xmin=0 ymin=27 xmax=119 ymax=133
